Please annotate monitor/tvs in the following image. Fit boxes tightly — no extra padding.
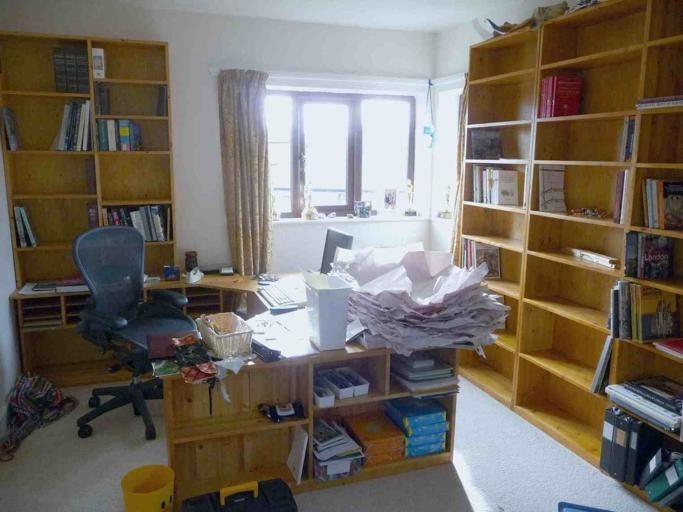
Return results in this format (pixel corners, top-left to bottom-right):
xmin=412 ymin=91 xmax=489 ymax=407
xmin=320 ymin=228 xmax=354 ymax=274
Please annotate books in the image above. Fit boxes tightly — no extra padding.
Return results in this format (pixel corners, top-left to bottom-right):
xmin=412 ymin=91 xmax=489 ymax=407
xmin=32 ymin=282 xmax=55 ymax=291
xmin=12 ymin=207 xmax=171 ymax=247
xmin=3 ymin=47 xmax=168 ymax=152
xmin=591 ymin=95 xmax=683 ymax=433
xmin=539 ymin=75 xmax=582 ymax=118
xmin=285 ymin=419 xmax=362 ymax=485
xmin=462 ymin=128 xmax=518 ymax=280
xmin=390 ymin=355 xmax=460 ymax=400
xmin=539 ymin=166 xmax=566 ymax=213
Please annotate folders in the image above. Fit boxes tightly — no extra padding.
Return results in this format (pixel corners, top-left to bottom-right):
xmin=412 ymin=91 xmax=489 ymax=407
xmin=600 ymin=407 xmax=683 ymax=502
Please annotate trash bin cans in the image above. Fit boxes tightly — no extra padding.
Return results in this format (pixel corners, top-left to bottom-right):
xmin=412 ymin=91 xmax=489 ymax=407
xmin=120 ymin=464 xmax=175 ymax=512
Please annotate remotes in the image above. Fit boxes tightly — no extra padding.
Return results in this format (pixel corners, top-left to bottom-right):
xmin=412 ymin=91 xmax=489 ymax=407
xmin=270 ymin=305 xmax=297 ymax=312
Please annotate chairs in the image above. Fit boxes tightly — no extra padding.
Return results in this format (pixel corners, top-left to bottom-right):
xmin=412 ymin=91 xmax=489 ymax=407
xmin=74 ymin=225 xmax=198 ymax=440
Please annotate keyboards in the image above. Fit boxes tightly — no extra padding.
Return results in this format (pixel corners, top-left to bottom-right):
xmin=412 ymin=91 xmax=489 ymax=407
xmin=262 ymin=284 xmax=296 ymax=306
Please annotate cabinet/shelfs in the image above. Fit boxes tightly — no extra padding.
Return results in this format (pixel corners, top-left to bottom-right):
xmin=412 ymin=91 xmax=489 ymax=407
xmin=1 ymin=30 xmax=177 ymax=289
xmin=455 ymin=1 xmax=682 ymax=512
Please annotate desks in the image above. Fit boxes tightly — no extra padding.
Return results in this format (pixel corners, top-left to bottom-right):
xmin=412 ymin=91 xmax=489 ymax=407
xmin=8 ymin=269 xmax=456 ymax=512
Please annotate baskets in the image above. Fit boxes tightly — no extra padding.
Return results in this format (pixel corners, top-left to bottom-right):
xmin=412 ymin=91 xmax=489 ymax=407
xmin=196 ymin=312 xmax=253 ymax=359
